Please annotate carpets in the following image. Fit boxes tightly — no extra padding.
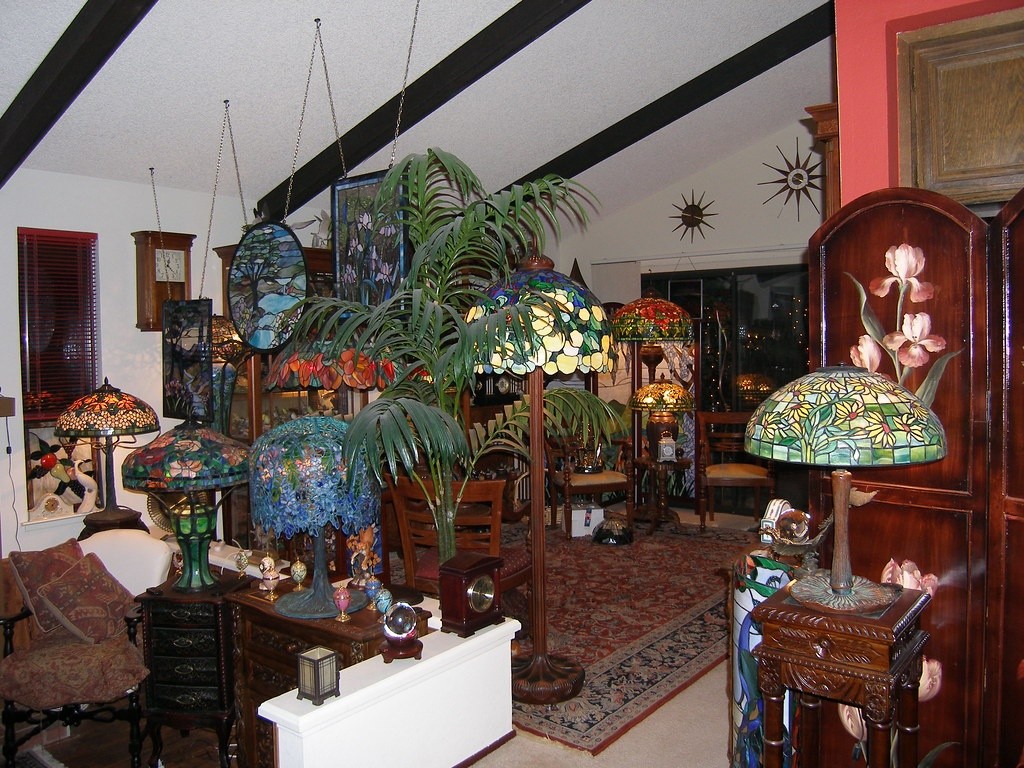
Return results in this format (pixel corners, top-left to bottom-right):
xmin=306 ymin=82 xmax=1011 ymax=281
xmin=369 ymin=521 xmax=788 ymax=757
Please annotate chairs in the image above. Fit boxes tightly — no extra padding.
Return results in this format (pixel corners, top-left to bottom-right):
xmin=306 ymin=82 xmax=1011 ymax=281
xmin=700 ymin=406 xmax=776 ymax=525
xmin=0 ymin=558 xmax=143 ymax=768
xmin=542 ymin=418 xmax=629 ymax=540
xmin=387 ymin=473 xmax=535 ymax=657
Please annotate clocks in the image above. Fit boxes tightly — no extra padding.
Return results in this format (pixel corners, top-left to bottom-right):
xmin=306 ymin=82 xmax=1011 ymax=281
xmin=132 ymin=230 xmax=197 ymax=331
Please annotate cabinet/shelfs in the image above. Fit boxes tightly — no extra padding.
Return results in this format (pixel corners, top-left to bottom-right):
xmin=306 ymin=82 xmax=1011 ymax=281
xmin=260 ymin=618 xmax=521 ymax=768
xmin=132 ymin=581 xmax=242 ymax=768
xmin=227 ymin=574 xmax=432 ymax=768
xmin=216 ymin=245 xmax=337 ymax=441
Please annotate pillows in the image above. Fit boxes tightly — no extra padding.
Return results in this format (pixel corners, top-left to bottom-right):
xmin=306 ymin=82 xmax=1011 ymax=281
xmin=9 ymin=538 xmax=85 ymax=635
xmin=37 ymin=552 xmax=138 ymax=644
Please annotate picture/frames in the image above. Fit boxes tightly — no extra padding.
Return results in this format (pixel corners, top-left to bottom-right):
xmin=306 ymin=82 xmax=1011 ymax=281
xmin=329 ymin=166 xmax=412 ymax=327
xmin=226 ymin=221 xmax=302 ymax=352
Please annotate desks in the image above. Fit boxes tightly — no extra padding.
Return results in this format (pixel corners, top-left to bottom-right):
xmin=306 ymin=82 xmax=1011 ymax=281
xmin=632 ymin=459 xmax=695 ymax=534
xmin=750 ymin=580 xmax=933 ymax=768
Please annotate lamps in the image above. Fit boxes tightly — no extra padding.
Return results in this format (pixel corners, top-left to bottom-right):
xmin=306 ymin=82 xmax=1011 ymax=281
xmin=54 ymin=375 xmax=162 ymax=526
xmin=249 ymin=417 xmax=375 ymax=620
xmin=611 ymin=298 xmax=699 ymax=522
xmin=462 ymin=269 xmax=617 ymax=705
xmin=123 ymin=415 xmax=256 ymax=592
xmin=745 ymin=362 xmax=948 ymax=618
xmin=629 ymin=382 xmax=695 ymax=457
